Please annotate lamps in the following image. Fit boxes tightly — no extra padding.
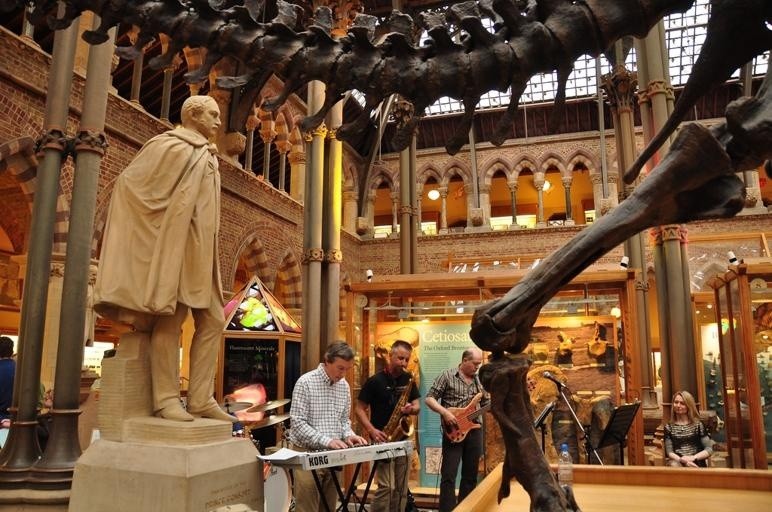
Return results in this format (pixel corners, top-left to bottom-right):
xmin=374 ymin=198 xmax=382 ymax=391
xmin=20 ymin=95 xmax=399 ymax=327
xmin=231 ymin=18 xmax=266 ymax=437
xmin=428 ymin=188 xmax=440 ymax=200
xmin=541 ymin=180 xmax=550 ymax=191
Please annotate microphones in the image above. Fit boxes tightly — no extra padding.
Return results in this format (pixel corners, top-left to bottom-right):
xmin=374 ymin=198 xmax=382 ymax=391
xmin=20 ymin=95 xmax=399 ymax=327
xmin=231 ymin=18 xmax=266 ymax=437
xmin=543 ymin=371 xmax=566 ymax=387
xmin=471 ymin=373 xmax=478 ymax=385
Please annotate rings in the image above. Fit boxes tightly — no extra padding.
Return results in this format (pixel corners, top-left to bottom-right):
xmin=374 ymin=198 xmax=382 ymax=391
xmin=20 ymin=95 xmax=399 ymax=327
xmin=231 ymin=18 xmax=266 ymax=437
xmin=375 ymin=436 xmax=379 ymax=442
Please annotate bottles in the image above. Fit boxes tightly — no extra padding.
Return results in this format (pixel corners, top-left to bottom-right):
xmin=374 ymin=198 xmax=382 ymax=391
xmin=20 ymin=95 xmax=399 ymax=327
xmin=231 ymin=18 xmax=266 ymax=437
xmin=558 ymin=443 xmax=574 ymax=490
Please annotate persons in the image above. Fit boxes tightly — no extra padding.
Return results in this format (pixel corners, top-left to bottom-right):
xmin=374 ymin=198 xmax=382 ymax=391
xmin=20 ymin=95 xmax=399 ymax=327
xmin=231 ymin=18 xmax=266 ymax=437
xmin=355 ymin=340 xmax=421 ymax=512
xmin=664 ymin=390 xmax=713 ymax=468
xmin=288 ymin=340 xmax=368 ymax=512
xmin=0 ymin=336 xmax=16 ymax=425
xmin=89 ymin=94 xmax=240 ymax=425
xmin=424 ymin=348 xmax=491 ymax=512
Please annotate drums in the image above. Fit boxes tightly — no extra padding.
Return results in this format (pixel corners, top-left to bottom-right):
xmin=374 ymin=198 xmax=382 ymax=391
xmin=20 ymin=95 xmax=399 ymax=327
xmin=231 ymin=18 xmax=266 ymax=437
xmin=263 ymin=461 xmax=292 ymax=512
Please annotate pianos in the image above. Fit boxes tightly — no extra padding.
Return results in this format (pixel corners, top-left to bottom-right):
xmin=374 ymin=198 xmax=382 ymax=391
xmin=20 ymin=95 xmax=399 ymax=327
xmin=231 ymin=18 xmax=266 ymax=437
xmin=269 ymin=440 xmax=414 ymax=472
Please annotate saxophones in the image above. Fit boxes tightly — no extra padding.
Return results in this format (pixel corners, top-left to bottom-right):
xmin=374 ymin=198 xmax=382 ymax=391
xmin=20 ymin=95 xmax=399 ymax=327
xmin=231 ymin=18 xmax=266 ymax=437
xmin=376 ymin=365 xmax=415 ymax=463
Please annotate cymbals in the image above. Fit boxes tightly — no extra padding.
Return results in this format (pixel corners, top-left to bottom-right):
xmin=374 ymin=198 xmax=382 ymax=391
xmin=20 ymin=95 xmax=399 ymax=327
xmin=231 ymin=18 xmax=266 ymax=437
xmin=247 ymin=399 xmax=290 ymax=412
xmin=218 ymin=401 xmax=251 ymax=413
xmin=250 ymin=414 xmax=290 ymax=429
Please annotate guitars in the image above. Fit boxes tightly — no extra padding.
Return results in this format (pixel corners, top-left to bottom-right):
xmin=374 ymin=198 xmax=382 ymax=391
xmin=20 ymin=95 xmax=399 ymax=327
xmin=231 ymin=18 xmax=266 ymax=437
xmin=441 ymin=393 xmax=491 ymax=443
